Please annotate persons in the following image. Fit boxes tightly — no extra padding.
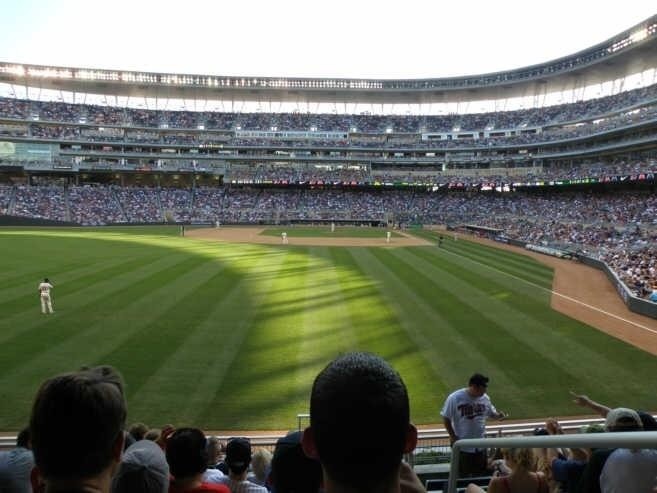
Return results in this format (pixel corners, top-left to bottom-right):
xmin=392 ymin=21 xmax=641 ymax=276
xmin=38 ymin=277 xmax=54 ymax=316
xmin=1 ymin=85 xmax=657 ymax=304
xmin=1 ymin=351 xmax=656 ymax=492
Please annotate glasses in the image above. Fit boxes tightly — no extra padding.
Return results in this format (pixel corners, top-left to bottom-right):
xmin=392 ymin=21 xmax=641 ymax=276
xmin=227 ymin=438 xmax=251 ymax=443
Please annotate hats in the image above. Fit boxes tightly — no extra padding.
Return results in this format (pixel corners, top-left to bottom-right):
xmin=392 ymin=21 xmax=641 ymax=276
xmin=606 ymin=408 xmax=644 ymax=429
xmin=119 ymin=439 xmax=169 ymax=493
xmin=227 ymin=440 xmax=251 ymax=467
